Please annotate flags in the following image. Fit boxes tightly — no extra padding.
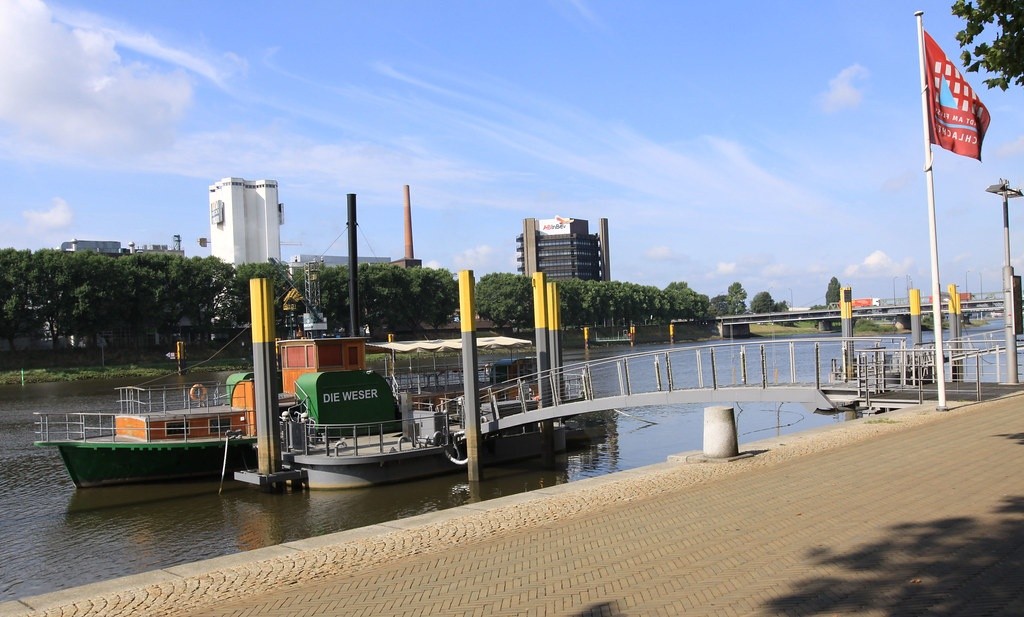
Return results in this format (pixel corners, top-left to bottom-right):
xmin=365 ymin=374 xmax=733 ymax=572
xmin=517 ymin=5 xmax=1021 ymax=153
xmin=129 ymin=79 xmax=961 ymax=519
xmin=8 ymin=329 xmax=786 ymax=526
xmin=921 ymin=22 xmax=990 ymax=162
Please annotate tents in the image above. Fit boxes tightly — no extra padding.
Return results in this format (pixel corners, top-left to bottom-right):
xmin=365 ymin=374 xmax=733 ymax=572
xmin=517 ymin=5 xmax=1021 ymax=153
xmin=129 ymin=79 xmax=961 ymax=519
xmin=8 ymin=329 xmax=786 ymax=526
xmin=366 ymin=336 xmax=534 ymax=390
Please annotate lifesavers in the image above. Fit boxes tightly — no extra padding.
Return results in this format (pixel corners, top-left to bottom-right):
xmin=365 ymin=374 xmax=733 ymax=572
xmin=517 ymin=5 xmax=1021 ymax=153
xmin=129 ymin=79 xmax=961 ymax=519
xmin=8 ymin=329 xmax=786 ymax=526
xmin=190 ymin=384 xmax=206 ymax=401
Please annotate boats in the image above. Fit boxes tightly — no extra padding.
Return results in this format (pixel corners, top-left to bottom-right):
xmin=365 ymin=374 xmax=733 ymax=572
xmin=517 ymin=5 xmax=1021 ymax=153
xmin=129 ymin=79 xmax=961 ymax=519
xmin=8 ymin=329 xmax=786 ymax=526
xmin=30 ymin=260 xmax=587 ymax=490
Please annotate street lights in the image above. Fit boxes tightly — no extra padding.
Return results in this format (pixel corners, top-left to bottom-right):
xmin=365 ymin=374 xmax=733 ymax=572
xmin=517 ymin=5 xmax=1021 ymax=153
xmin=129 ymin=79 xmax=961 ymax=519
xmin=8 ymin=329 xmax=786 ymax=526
xmin=984 ymin=177 xmax=1024 ymax=386
